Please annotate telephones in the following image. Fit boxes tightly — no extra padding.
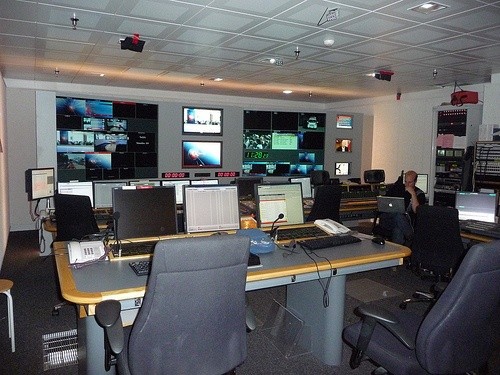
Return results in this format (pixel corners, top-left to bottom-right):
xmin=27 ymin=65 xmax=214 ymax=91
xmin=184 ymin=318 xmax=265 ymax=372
xmin=40 ymin=208 xmax=56 ymax=220
xmin=67 ymin=240 xmax=109 ymax=266
xmin=313 ymin=218 xmax=351 ymax=236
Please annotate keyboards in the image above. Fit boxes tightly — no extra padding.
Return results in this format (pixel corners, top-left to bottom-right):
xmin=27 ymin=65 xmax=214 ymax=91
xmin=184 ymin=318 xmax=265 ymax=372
xmin=128 ymin=260 xmax=152 ymax=276
xmin=264 ymin=226 xmax=328 ymax=241
xmin=95 ymin=215 xmax=112 ymax=220
xmin=305 ymin=234 xmax=361 ymax=250
xmin=110 ymin=240 xmax=157 ymax=258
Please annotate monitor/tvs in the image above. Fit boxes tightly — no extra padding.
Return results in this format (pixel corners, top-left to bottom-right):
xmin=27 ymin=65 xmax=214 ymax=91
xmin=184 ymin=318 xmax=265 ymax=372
xmin=336 ymin=115 xmax=354 ymax=129
xmin=403 ymin=174 xmax=428 ymax=194
xmin=25 ymin=167 xmax=313 ymax=241
xmin=182 ymin=140 xmax=223 ymax=167
xmin=336 ymin=138 xmax=352 ymax=152
xmin=335 ymin=162 xmax=350 ymax=176
xmin=182 ymin=106 xmax=223 ymax=136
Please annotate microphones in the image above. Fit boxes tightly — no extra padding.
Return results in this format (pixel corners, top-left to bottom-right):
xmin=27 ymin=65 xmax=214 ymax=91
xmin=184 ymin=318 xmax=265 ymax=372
xmin=270 ymin=213 xmax=284 ymax=238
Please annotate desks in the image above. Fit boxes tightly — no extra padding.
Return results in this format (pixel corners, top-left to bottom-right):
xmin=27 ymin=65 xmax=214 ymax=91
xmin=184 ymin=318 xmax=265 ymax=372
xmin=44 ymin=198 xmax=500 ymax=375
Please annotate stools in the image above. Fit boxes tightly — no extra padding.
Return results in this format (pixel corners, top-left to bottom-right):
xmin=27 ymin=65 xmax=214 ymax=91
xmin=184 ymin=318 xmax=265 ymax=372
xmin=0 ymin=279 xmax=18 ymax=352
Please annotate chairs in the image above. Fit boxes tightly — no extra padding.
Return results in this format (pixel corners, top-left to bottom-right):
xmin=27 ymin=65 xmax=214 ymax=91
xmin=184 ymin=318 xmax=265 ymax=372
xmin=399 ymin=204 xmax=467 ymax=310
xmin=95 ymin=235 xmax=250 ymax=375
xmin=52 ymin=194 xmax=102 ymax=317
xmin=342 ymin=241 xmax=500 ymax=375
xmin=306 ymin=185 xmax=343 ymax=226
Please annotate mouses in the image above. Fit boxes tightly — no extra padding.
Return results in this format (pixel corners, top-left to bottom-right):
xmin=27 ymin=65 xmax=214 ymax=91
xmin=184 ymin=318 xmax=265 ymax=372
xmin=372 ymin=237 xmax=385 ymax=245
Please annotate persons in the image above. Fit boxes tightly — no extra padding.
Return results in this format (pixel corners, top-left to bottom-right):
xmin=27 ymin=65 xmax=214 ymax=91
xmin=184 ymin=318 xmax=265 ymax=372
xmin=378 ymin=170 xmax=426 ymax=272
xmin=336 ymin=141 xmax=349 ymax=152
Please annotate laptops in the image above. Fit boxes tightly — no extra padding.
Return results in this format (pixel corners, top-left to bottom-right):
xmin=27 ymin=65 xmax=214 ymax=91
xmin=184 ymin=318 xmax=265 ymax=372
xmin=376 ymin=196 xmax=406 ymax=214
xmin=454 ymin=191 xmax=497 ymax=224
